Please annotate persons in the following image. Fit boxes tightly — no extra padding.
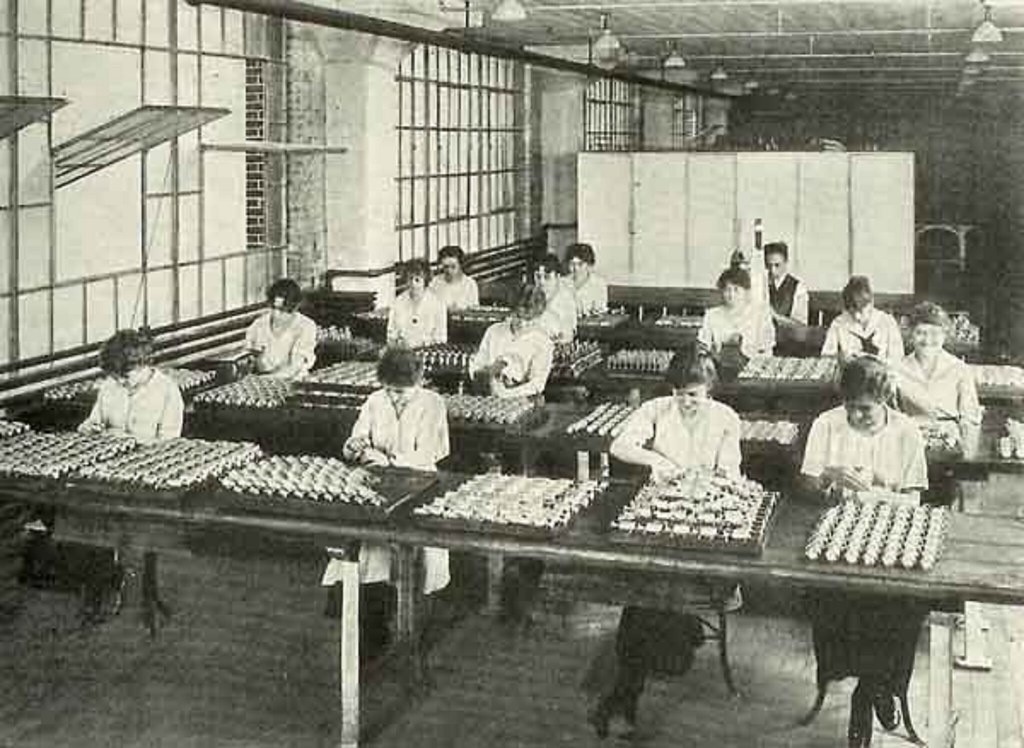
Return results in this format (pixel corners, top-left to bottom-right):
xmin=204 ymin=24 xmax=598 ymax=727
xmin=465 ymin=284 xmax=555 ymax=403
xmin=763 ymin=242 xmax=810 ymax=328
xmin=888 ymin=303 xmax=982 ymax=455
xmin=430 ymin=245 xmax=480 ymax=310
xmin=79 ymin=329 xmax=184 ymax=618
xmin=535 ymin=252 xmax=578 ymax=345
xmin=609 ymin=339 xmax=742 ymax=673
xmin=797 ymin=355 xmax=928 ymax=731
xmin=320 ymin=346 xmax=453 ymax=658
xmin=562 ymin=244 xmax=607 ymax=318
xmin=386 ymin=259 xmax=447 ymax=350
xmin=696 ymin=266 xmax=776 ymax=359
xmin=820 ymin=274 xmax=906 ymax=362
xmin=245 ymin=278 xmax=317 ymax=381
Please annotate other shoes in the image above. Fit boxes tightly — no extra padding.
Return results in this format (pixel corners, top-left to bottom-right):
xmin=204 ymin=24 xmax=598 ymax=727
xmin=82 ymin=549 xmax=118 ymax=615
xmin=92 ymin=561 xmax=125 ymax=621
xmin=849 ymin=686 xmax=873 ymax=748
xmin=875 ymin=692 xmax=903 ymax=732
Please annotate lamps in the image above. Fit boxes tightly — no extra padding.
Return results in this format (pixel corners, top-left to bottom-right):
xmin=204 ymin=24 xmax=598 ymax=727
xmin=710 ymin=64 xmax=728 ymax=81
xmin=970 ymin=8 xmax=1003 ymax=43
xmin=591 ymin=29 xmax=625 ymax=71
xmin=664 ymin=48 xmax=686 ymax=70
xmin=966 ymin=46 xmax=991 ymax=63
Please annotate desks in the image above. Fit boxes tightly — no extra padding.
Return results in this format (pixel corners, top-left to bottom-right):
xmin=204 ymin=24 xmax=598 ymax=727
xmin=1 ymin=453 xmax=1024 ymax=747
xmin=346 ymin=307 xmax=986 ymax=362
xmin=478 ymin=282 xmax=960 ymax=327
xmin=314 ymin=338 xmax=1024 ymax=512
xmin=30 ymin=364 xmax=1024 ymax=671
